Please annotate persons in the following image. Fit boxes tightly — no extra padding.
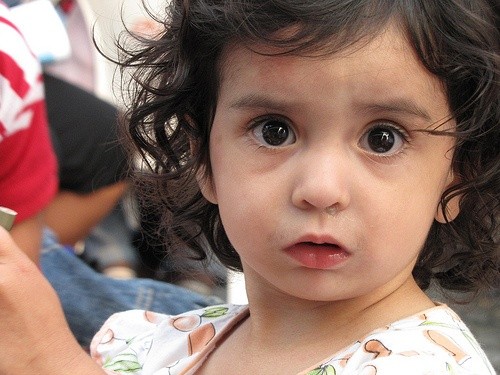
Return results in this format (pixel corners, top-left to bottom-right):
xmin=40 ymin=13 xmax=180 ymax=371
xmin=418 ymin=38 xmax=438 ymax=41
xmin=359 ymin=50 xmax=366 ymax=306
xmin=0 ymin=0 xmax=229 ymax=352
xmin=0 ymin=0 xmax=500 ymax=375
